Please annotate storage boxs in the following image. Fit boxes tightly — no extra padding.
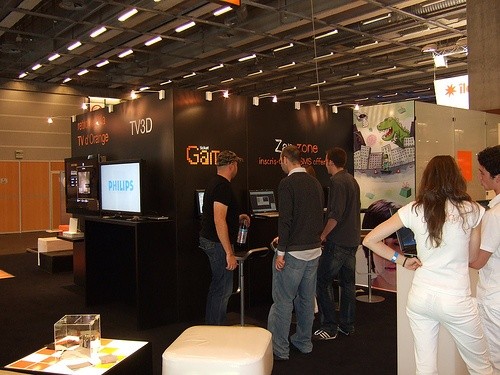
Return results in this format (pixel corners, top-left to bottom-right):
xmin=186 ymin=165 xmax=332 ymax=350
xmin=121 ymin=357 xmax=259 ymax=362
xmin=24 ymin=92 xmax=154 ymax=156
xmin=53 ymin=314 xmax=101 ymax=359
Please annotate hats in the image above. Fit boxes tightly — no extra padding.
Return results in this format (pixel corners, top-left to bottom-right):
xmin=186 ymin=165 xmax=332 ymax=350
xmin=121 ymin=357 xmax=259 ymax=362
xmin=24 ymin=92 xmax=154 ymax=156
xmin=217 ymin=151 xmax=242 ymax=166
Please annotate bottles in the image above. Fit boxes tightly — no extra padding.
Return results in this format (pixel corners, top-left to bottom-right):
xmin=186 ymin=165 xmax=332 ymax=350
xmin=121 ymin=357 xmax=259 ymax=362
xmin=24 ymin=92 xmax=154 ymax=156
xmin=234 ymin=220 xmax=247 ymax=254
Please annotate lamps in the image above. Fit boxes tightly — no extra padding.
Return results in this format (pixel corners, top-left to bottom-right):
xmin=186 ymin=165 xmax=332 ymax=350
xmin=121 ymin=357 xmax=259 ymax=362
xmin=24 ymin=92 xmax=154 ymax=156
xmin=253 ymin=95 xmax=278 ymax=106
xmin=420 ymin=43 xmax=438 ymax=54
xmin=82 ymin=102 xmax=113 ymax=113
xmin=295 ymin=100 xmax=320 ymax=110
xmin=130 ymin=90 xmax=166 ymax=100
xmin=434 ymin=54 xmax=449 ymax=69
xmin=48 ymin=115 xmax=76 ymax=124
xmin=332 ymin=104 xmax=360 ymax=113
xmin=206 ymin=90 xmax=228 ymax=101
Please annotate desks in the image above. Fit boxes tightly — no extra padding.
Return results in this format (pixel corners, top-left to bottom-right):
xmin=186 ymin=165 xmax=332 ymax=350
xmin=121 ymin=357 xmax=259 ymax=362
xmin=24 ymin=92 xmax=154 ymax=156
xmin=0 ymin=338 xmax=154 ymax=375
xmin=83 ymin=214 xmax=177 ymax=329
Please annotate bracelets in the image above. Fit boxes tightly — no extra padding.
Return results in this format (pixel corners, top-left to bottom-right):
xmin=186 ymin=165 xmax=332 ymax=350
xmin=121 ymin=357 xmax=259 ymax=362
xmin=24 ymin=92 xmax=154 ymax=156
xmin=391 ymin=252 xmax=398 ymax=263
xmin=403 ymin=257 xmax=408 ymax=267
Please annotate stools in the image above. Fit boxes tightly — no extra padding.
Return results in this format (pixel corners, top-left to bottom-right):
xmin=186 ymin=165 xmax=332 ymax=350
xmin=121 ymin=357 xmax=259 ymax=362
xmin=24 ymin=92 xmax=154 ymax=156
xmin=231 ymin=245 xmax=269 ymax=326
xmin=355 ymin=228 xmax=386 ymax=303
xmin=161 ymin=324 xmax=273 ymax=375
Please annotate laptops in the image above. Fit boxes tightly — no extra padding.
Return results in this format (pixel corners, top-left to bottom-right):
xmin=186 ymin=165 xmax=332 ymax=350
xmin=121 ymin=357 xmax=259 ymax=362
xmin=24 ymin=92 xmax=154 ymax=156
xmin=388 ymin=207 xmax=417 ymax=256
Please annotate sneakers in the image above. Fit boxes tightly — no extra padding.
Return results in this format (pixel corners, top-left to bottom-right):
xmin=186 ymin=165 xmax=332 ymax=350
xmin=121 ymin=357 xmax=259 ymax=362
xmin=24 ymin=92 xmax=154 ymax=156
xmin=337 ymin=326 xmax=353 ymax=336
xmin=312 ymin=329 xmax=337 ymax=340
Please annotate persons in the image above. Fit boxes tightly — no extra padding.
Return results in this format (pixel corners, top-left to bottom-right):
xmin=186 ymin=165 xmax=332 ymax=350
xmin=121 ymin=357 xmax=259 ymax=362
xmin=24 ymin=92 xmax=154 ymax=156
xmin=314 ymin=147 xmax=361 ymax=340
xmin=363 ymin=155 xmax=494 ymax=375
xmin=198 ymin=152 xmax=250 ymax=326
xmin=267 ymin=145 xmax=323 ymax=362
xmin=469 ymin=145 xmax=500 ymax=375
xmin=361 ymin=199 xmax=403 ymax=291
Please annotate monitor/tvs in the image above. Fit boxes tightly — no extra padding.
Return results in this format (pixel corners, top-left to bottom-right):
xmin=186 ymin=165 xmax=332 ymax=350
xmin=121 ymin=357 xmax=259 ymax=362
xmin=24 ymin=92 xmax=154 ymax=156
xmin=249 ymin=189 xmax=277 ymax=212
xmin=97 ymin=160 xmax=144 ymax=216
